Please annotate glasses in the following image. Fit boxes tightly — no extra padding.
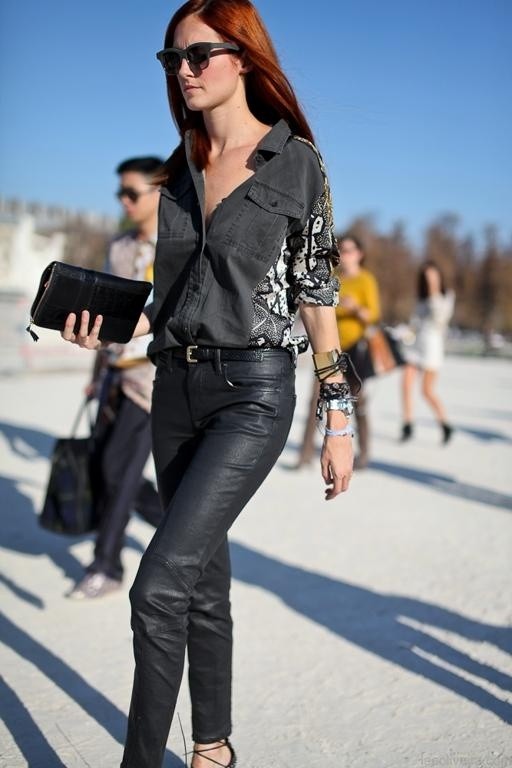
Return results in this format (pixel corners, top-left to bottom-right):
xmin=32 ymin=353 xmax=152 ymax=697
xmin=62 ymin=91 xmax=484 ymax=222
xmin=117 ymin=189 xmax=157 ymax=203
xmin=156 ymin=42 xmax=241 ymax=72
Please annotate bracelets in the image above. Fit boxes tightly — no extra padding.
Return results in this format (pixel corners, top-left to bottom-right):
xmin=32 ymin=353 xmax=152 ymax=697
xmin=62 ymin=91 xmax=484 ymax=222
xmin=312 ymin=349 xmax=363 ymax=437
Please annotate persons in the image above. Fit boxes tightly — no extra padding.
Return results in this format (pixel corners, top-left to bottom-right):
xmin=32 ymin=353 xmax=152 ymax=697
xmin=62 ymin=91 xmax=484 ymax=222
xmin=379 ymin=258 xmax=454 ymax=446
xmin=284 ymin=235 xmax=382 ymax=471
xmin=59 ymin=0 xmax=357 ymax=768
xmin=62 ymin=156 xmax=168 ymax=604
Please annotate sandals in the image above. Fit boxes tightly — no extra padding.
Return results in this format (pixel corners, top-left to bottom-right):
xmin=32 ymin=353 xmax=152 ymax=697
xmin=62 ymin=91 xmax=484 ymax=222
xmin=191 ymin=737 xmax=234 ymax=768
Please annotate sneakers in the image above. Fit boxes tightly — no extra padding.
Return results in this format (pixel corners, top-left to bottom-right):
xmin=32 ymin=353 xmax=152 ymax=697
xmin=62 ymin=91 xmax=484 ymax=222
xmin=401 ymin=425 xmax=453 ymax=442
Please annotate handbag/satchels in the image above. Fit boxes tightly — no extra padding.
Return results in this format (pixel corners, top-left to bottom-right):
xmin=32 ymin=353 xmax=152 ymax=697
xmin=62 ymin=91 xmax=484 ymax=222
xmin=39 ymin=439 xmax=103 ymax=535
xmin=342 ymin=325 xmax=404 ymax=380
xmin=29 ymin=261 xmax=152 ymax=343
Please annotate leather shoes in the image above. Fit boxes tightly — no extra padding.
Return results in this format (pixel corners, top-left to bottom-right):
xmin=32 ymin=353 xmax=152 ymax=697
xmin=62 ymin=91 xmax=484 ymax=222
xmin=68 ymin=570 xmax=120 ymax=599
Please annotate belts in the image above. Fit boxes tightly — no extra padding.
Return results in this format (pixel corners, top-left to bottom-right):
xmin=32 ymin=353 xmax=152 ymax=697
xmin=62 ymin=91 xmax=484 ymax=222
xmin=154 ymin=346 xmax=289 ymax=362
